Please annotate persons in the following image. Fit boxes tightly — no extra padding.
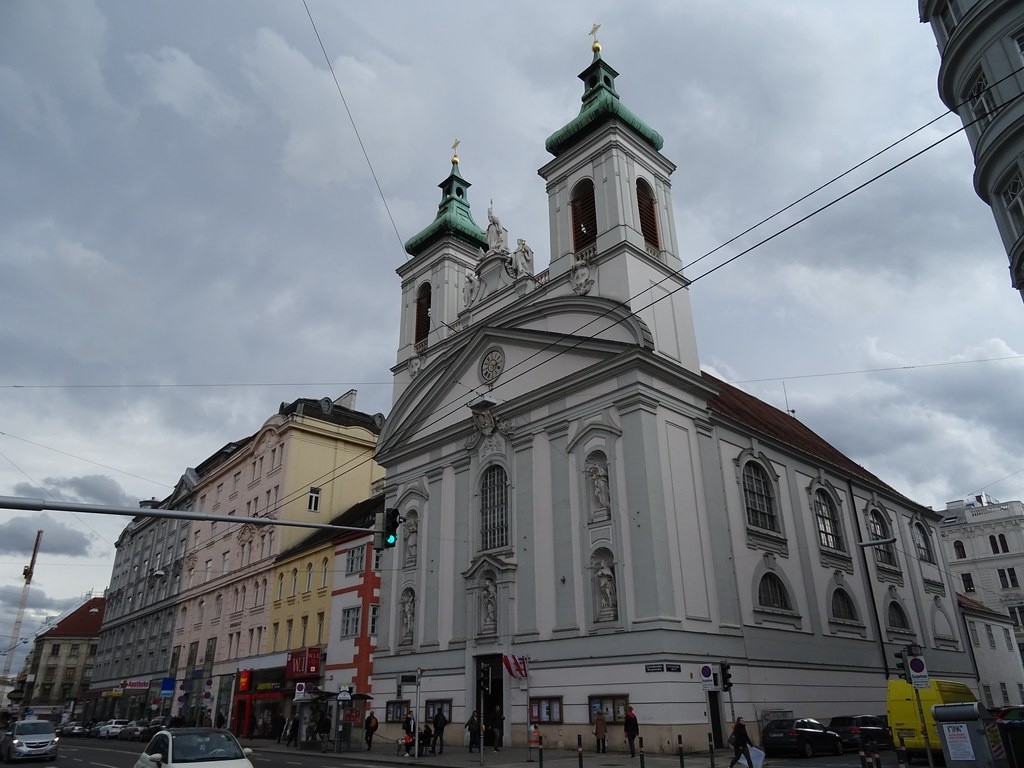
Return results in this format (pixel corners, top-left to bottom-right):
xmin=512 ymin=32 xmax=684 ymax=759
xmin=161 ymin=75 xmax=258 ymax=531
xmin=729 ymin=716 xmax=754 ymax=768
xmin=428 ymin=708 xmax=447 ymax=754
xmin=512 ymin=238 xmax=532 ymax=280
xmin=275 ymin=712 xmax=299 ymax=747
xmin=484 ymin=198 xmax=501 ymax=252
xmin=465 ymin=711 xmax=482 ymax=754
xmin=305 ymin=705 xmax=326 ymax=741
xmin=463 ymin=270 xmax=479 ymax=306
xmin=593 ymin=709 xmax=607 ymax=753
xmin=319 ymin=713 xmax=335 ymax=753
xmin=197 ymin=707 xmax=212 ymax=727
xmin=365 ymin=711 xmax=378 ymax=751
xmin=216 ymin=711 xmax=225 ymax=728
xmin=489 ymin=704 xmax=505 ymax=753
xmin=623 ymin=706 xmax=639 ymax=758
xmin=404 ymin=711 xmax=432 ymax=756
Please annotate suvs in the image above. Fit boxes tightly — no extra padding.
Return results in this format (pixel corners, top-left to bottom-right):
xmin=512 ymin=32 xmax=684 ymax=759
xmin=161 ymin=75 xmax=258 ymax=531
xmin=991 ymin=704 xmax=1024 ymax=742
xmin=831 ymin=714 xmax=894 ymax=749
xmin=5 ymin=720 xmax=62 ymax=761
xmin=762 ymin=716 xmax=846 ymax=757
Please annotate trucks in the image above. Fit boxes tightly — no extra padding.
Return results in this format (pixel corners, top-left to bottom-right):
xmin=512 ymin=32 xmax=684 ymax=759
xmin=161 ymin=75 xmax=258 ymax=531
xmin=885 ymin=677 xmax=982 ymax=762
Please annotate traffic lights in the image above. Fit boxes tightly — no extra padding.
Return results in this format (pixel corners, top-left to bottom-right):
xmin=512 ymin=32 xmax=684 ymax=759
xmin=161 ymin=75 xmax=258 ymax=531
xmin=480 ymin=666 xmax=491 ymax=695
xmin=894 ymin=650 xmax=916 ymax=685
xmin=374 ymin=507 xmax=400 ymax=550
xmin=717 ymin=660 xmax=734 ymax=693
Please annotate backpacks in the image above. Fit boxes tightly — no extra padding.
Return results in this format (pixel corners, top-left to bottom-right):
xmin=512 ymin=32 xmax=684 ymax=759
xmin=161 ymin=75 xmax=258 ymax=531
xmin=370 ymin=716 xmax=376 ymax=727
xmin=438 ymin=713 xmax=445 ymax=727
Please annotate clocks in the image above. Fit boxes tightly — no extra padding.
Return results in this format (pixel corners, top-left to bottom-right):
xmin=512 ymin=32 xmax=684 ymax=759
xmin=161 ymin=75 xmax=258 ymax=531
xmin=477 ymin=344 xmax=505 ymax=386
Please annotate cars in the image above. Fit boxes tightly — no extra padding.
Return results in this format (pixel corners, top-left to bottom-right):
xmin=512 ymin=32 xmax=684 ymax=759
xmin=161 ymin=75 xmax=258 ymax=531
xmin=132 ymin=727 xmax=254 ymax=768
xmin=56 ymin=715 xmax=187 ymax=742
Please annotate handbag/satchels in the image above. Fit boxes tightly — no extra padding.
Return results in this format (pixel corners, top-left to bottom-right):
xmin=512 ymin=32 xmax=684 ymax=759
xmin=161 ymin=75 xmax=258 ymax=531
xmin=592 ymin=724 xmax=596 ymax=735
xmin=728 ymin=733 xmax=736 ymax=746
xmin=605 ymin=734 xmax=608 ymax=747
